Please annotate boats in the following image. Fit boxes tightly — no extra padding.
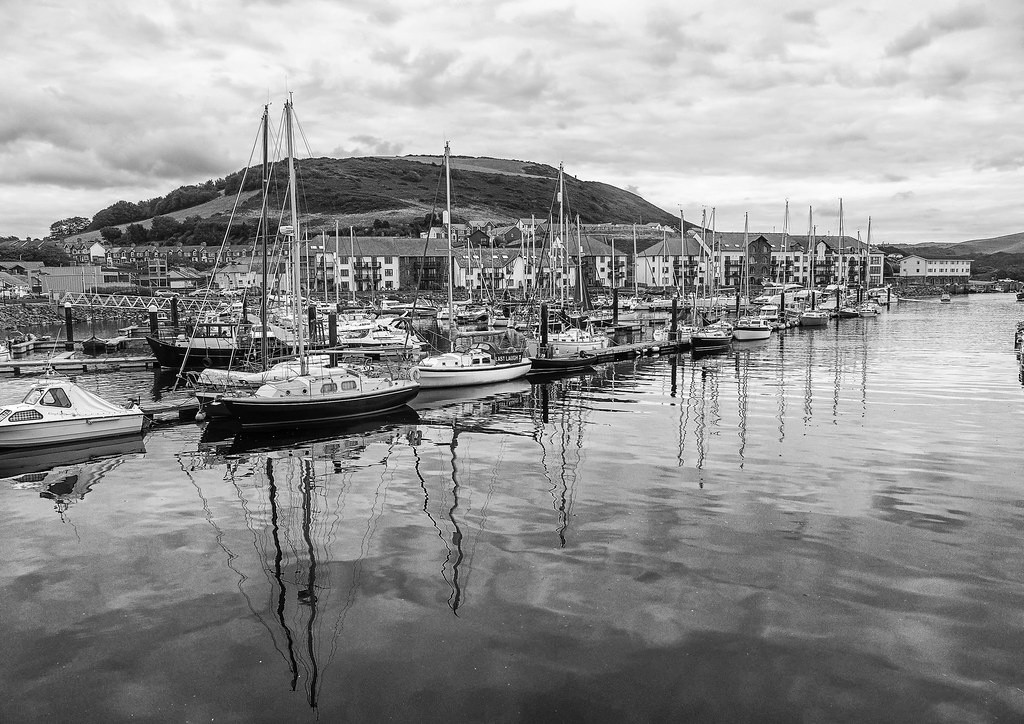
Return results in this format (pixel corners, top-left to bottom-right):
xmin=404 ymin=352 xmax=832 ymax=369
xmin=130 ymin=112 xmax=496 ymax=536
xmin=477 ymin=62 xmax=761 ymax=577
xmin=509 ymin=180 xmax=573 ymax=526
xmin=1 ymin=378 xmax=144 ymax=448
xmin=941 ymin=293 xmax=951 ymax=302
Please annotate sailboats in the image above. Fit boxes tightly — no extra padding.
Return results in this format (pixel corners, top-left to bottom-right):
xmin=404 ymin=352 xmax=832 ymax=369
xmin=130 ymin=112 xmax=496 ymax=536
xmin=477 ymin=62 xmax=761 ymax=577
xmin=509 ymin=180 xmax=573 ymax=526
xmin=732 ymin=197 xmax=898 ymax=344
xmin=146 ymin=91 xmax=735 ymax=429
